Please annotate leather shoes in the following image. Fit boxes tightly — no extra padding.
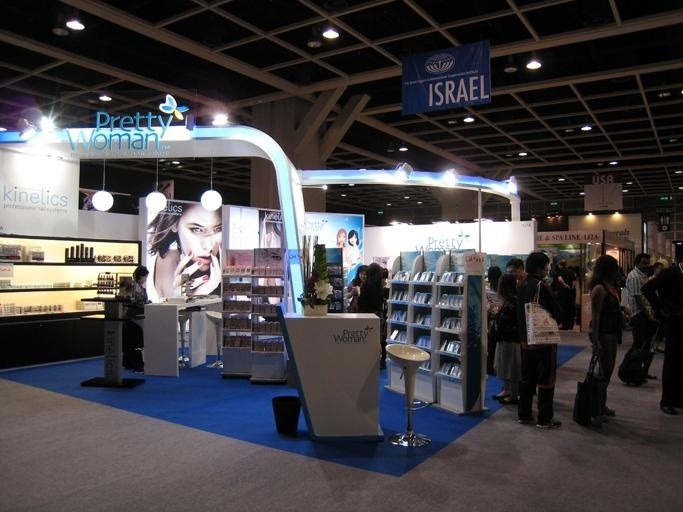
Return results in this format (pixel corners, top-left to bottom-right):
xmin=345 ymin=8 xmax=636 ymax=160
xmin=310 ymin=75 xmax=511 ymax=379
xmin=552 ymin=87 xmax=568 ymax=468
xmin=644 ymin=373 xmax=658 ymax=379
xmin=659 ymin=406 xmax=676 ymax=414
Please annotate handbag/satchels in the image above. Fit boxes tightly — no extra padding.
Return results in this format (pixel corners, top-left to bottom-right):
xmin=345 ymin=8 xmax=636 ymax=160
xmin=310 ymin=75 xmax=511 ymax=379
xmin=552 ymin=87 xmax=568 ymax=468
xmin=573 ymin=372 xmax=607 ymax=421
xmin=489 ymin=312 xmax=522 ymax=343
xmin=525 ymin=302 xmax=561 ymax=345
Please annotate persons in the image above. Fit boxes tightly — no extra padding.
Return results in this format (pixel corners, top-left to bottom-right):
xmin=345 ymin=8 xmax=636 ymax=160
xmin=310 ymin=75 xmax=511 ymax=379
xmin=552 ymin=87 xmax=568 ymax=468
xmin=486 ymin=252 xmax=683 ymax=428
xmin=255 ymin=248 xmax=285 ymax=304
xmin=147 ymin=201 xmax=223 ymax=300
xmin=346 ymin=263 xmax=387 ymax=368
xmin=118 ymin=265 xmax=152 ymax=374
xmin=337 ymin=228 xmax=361 ymax=267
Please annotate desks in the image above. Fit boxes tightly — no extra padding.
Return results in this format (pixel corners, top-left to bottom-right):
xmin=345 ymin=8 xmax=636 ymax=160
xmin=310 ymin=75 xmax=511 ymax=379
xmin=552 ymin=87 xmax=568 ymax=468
xmin=144 ymin=294 xmax=223 ymax=377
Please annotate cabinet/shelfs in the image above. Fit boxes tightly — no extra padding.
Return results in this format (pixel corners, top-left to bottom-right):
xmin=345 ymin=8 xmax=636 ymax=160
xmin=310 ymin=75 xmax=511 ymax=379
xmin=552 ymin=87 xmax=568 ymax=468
xmin=324 ymin=249 xmax=343 ymax=313
xmin=249 ymin=267 xmax=289 ymax=383
xmin=220 ymin=270 xmax=252 ymax=376
xmin=385 ymin=252 xmax=489 ymax=416
xmin=0 ymin=233 xmax=142 ymax=372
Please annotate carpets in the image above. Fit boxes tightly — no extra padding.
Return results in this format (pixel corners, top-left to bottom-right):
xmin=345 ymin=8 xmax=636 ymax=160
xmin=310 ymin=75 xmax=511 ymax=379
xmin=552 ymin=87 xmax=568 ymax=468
xmin=0 ymin=344 xmax=587 ymax=477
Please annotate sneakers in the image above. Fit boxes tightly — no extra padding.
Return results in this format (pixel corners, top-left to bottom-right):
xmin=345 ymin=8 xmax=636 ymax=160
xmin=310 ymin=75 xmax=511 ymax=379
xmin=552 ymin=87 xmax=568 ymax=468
xmin=601 ymin=414 xmax=608 ymax=422
xmin=603 ymin=406 xmax=615 ymax=415
xmin=492 ymin=393 xmax=562 ymax=427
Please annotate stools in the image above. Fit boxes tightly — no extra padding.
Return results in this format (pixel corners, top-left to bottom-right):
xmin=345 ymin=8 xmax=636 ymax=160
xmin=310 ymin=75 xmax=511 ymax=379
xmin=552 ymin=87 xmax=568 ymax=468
xmin=205 ymin=310 xmax=223 ymax=369
xmin=166 ymin=297 xmax=190 ymax=370
xmin=385 ymin=343 xmax=437 ymax=449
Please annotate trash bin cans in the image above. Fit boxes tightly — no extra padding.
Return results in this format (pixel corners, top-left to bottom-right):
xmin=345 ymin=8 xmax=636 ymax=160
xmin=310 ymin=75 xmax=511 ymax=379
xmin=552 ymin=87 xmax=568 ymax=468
xmin=272 ymin=396 xmax=301 ymax=435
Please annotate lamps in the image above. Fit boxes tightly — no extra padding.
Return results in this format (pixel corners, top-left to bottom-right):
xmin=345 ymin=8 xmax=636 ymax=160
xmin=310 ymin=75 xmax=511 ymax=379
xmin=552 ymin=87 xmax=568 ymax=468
xmin=386 ymin=139 xmax=395 ymax=153
xmin=397 ymin=141 xmax=409 ymax=152
xmin=91 ymin=160 xmax=114 ymax=212
xmin=448 ymin=118 xmax=457 ymax=125
xmin=306 ymin=28 xmax=323 ymax=48
xmin=98 ymin=93 xmax=112 ymax=101
xmin=526 ymin=51 xmax=543 ymax=70
xmin=322 ymin=19 xmax=340 ymax=40
xmin=463 ymin=111 xmax=475 ymax=123
xmin=65 ymin=9 xmax=86 ymax=31
xmin=145 ymin=159 xmax=168 ymax=211
xmin=200 ymin=157 xmax=223 ymax=212
